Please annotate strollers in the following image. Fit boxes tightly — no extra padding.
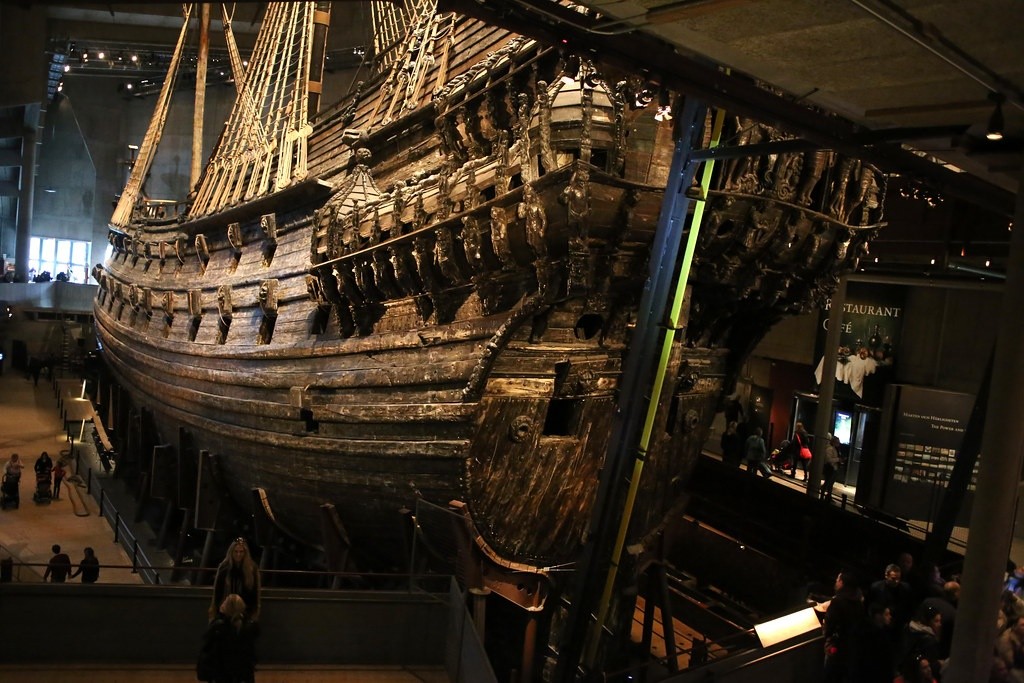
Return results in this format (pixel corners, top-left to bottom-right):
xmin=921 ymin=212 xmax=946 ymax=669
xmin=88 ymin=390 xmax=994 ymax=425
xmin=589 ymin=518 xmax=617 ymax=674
xmin=765 ymin=439 xmax=792 ymax=473
xmin=33 ymin=468 xmax=53 ymax=504
xmin=0 ymin=472 xmax=21 ymax=510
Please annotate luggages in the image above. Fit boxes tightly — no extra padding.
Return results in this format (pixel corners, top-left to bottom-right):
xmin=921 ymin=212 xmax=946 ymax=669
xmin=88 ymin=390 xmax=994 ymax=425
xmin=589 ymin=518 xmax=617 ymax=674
xmin=759 ymin=464 xmax=773 ymax=478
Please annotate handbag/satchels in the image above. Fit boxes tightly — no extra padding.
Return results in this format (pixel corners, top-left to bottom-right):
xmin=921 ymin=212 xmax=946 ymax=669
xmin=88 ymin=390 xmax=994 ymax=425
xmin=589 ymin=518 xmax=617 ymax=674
xmin=799 ymin=447 xmax=813 ymax=459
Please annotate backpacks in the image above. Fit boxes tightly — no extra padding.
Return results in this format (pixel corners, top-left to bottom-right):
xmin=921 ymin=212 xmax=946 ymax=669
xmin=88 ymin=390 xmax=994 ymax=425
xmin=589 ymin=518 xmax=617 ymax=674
xmin=197 ymin=632 xmax=221 ymax=681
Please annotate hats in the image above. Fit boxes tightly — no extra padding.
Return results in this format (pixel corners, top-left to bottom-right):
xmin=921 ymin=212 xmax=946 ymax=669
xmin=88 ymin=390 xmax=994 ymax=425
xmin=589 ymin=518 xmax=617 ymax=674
xmin=220 ymin=594 xmax=247 ymax=615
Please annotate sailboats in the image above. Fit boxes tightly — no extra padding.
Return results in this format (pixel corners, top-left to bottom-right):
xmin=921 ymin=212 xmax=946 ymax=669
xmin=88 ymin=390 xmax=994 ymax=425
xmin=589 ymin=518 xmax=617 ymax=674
xmin=85 ymin=0 xmax=893 ymax=682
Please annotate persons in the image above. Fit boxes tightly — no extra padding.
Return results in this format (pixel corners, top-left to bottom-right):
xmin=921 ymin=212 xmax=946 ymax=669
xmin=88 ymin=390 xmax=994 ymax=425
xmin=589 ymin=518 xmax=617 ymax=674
xmin=2 ymin=453 xmax=25 ymax=509
xmin=719 ymin=420 xmax=845 ymax=502
xmin=70 ymin=547 xmax=100 ymax=585
xmin=809 ymin=555 xmax=1024 ymax=683
xmin=34 ymin=452 xmax=54 ymax=494
xmin=42 ymin=545 xmax=73 ymax=586
xmin=208 ymin=538 xmax=260 ymax=646
xmin=49 ymin=457 xmax=66 ymax=499
xmin=23 ymin=362 xmax=52 ymax=385
xmin=196 ymin=593 xmax=257 ymax=683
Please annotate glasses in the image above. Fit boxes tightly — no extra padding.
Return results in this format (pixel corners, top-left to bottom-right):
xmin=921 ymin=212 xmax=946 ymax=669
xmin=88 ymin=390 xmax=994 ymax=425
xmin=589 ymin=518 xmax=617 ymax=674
xmin=915 ymin=654 xmax=922 ymax=661
xmin=234 ymin=538 xmax=247 ymax=542
